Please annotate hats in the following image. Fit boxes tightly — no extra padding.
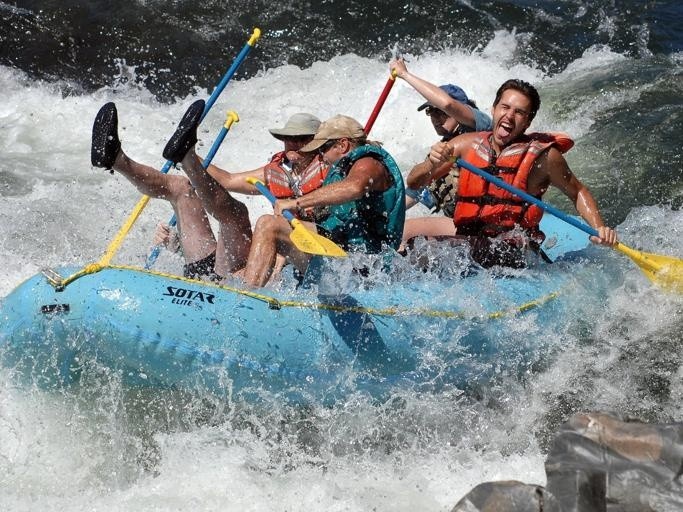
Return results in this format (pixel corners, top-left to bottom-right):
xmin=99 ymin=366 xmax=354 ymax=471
xmin=269 ymin=113 xmax=321 ymax=142
xmin=298 ymin=114 xmax=366 ymax=153
xmin=418 ymin=84 xmax=468 ymax=111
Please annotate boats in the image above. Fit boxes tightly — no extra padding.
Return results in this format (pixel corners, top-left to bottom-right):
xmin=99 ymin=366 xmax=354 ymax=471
xmin=0 ymin=207 xmax=632 ymax=419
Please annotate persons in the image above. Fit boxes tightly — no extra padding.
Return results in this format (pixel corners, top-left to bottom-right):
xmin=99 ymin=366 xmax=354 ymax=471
xmin=196 ymin=112 xmax=333 ymax=272
xmin=406 ymin=78 xmax=617 ymax=280
xmin=388 ymin=58 xmax=494 ymax=256
xmin=234 ymin=113 xmax=406 ymax=291
xmin=89 ymin=100 xmax=254 ymax=283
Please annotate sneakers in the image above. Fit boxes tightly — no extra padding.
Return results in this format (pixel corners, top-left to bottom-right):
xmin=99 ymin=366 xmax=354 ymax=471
xmin=91 ymin=103 xmax=119 ymax=170
xmin=163 ymin=98 xmax=205 ymax=164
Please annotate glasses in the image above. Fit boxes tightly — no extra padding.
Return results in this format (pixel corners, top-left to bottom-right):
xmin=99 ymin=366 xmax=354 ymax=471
xmin=319 ymin=141 xmax=336 ymax=154
xmin=283 ymin=135 xmax=312 ymax=142
xmin=426 ymin=108 xmax=444 ymax=116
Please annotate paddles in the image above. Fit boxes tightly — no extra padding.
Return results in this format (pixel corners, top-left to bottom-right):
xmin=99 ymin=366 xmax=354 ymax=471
xmin=245 ymin=176 xmax=347 ymax=258
xmin=455 ymin=158 xmax=683 ymax=293
xmin=97 ymin=27 xmax=262 ymax=263
